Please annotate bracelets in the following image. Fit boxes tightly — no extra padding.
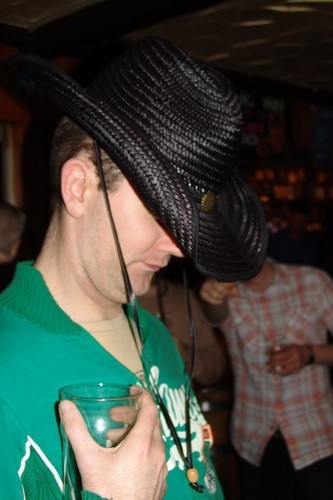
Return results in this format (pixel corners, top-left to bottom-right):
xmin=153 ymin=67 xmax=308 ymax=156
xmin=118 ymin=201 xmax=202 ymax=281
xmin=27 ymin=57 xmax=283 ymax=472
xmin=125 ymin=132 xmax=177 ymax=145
xmin=305 ymin=345 xmax=315 ymax=366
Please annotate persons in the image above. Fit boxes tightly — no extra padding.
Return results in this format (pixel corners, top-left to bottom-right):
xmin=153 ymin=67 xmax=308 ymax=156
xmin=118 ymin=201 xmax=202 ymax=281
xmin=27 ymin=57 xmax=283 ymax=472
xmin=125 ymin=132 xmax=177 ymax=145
xmin=136 ymin=274 xmax=225 ymax=384
xmin=199 ymin=258 xmax=333 ymax=500
xmin=0 ymin=202 xmax=26 ymax=290
xmin=0 ymin=38 xmax=268 ymax=500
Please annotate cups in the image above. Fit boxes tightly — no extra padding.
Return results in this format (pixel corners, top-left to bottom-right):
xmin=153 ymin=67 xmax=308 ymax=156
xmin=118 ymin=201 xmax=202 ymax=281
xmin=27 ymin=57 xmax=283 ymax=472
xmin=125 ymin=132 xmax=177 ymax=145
xmin=59 ymin=382 xmax=144 ymax=500
xmin=269 ymin=336 xmax=288 ymax=372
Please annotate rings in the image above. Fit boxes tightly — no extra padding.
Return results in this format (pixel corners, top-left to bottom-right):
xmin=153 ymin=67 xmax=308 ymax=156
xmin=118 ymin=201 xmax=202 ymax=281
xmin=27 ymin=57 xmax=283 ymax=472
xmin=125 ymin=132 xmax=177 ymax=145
xmin=275 ymin=365 xmax=283 ymax=371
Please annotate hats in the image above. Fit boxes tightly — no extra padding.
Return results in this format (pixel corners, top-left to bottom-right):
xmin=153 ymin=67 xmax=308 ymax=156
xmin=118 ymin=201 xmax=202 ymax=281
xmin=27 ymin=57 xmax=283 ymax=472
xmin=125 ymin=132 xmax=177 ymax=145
xmin=0 ymin=36 xmax=268 ymax=283
